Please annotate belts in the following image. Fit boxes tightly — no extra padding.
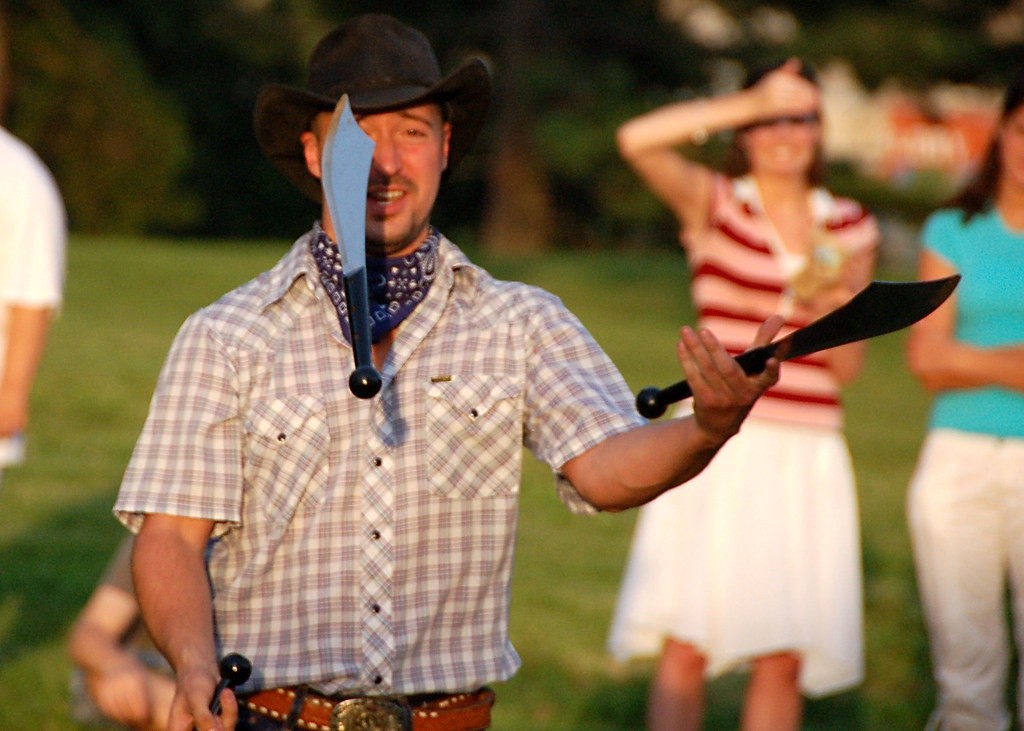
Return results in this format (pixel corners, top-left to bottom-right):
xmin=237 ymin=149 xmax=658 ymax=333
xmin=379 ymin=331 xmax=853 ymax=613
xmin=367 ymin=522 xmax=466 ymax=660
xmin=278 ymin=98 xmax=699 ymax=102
xmin=238 ymin=684 xmax=496 ymax=731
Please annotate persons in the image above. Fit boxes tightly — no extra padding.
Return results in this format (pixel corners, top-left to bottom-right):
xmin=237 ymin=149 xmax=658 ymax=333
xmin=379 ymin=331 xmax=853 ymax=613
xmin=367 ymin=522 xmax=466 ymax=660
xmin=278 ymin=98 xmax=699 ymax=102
xmin=902 ymin=79 xmax=1024 ymax=731
xmin=70 ymin=17 xmax=783 ymax=731
xmin=0 ymin=119 xmax=67 ymax=469
xmin=611 ymin=61 xmax=881 ymax=731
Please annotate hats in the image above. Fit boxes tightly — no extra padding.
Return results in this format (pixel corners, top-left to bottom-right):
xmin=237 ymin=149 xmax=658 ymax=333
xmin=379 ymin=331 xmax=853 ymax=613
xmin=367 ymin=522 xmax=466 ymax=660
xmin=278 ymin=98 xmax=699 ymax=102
xmin=252 ymin=12 xmax=492 ymax=205
